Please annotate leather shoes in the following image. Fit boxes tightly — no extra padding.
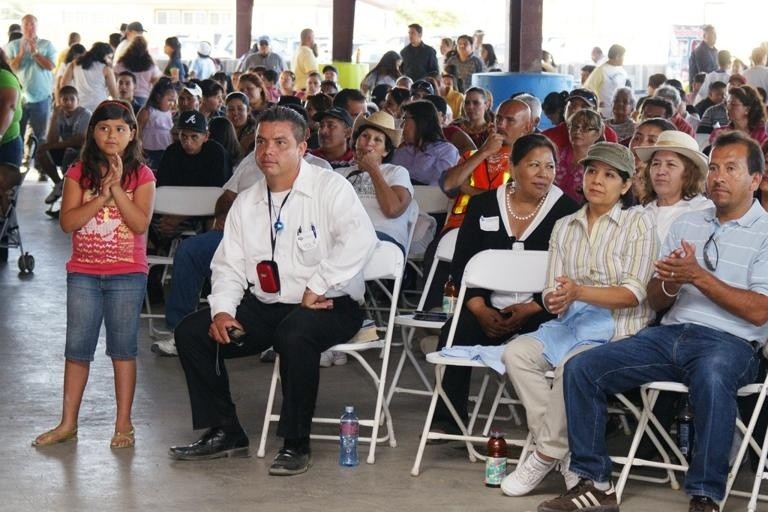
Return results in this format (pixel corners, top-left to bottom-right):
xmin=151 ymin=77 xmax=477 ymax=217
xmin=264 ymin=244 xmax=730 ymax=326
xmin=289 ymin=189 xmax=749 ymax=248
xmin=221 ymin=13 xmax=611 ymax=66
xmin=167 ymin=425 xmax=250 ymax=460
xmin=269 ymin=447 xmax=313 ymax=475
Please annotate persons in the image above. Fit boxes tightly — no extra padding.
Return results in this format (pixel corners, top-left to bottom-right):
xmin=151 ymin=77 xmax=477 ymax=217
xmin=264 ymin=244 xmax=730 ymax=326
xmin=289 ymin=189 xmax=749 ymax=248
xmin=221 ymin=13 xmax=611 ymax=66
xmin=172 ymin=107 xmax=380 ymax=473
xmin=417 ymin=22 xmax=767 ymax=512
xmin=1 ymin=10 xmax=441 ymax=367
xmin=33 ymin=100 xmax=158 ymax=447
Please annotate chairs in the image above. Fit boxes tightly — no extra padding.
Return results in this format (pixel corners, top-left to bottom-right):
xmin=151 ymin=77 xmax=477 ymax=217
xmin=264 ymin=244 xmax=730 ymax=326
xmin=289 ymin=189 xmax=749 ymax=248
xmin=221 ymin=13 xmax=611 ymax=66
xmin=380 ymin=200 xmax=418 ymax=360
xmin=359 ymin=184 xmax=450 ymax=359
xmin=377 ymin=229 xmax=459 ymax=426
xmin=138 ymin=186 xmax=224 ymax=340
xmin=411 ymin=248 xmax=554 ymax=476
xmin=257 ymin=240 xmax=404 ymax=464
xmin=612 ymin=380 xmax=767 ymax=510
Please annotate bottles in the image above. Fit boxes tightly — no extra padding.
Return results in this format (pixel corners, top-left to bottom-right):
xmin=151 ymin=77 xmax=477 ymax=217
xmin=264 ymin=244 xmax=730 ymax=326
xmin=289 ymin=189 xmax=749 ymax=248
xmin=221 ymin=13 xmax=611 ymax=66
xmin=441 ymin=276 xmax=458 ymax=315
xmin=339 ymin=405 xmax=359 ymax=467
xmin=486 ymin=428 xmax=507 ymax=488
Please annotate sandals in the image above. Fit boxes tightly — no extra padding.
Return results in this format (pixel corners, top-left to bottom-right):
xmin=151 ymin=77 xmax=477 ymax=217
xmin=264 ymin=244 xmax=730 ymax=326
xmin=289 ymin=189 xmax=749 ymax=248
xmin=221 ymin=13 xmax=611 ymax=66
xmin=32 ymin=424 xmax=79 ymax=447
xmin=110 ymin=426 xmax=136 ymax=449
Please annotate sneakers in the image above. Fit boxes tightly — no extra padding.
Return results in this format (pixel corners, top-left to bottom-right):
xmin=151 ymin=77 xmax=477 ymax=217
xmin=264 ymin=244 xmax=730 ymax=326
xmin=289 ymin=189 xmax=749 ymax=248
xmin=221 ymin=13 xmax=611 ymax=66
xmin=555 ymin=451 xmax=581 ymax=492
xmin=46 ymin=211 xmax=59 ymax=221
xmin=501 ymin=453 xmax=558 ymax=497
xmin=688 ymin=496 xmax=719 ymax=512
xmin=150 ymin=338 xmax=350 ymax=368
xmin=45 ymin=180 xmax=63 ymax=203
xmin=537 ymin=475 xmax=620 ymax=512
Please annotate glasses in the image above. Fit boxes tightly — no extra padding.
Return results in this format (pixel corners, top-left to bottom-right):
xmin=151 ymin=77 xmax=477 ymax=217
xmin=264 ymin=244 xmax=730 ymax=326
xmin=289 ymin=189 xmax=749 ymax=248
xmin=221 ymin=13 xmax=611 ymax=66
xmin=567 ymin=124 xmax=599 ymax=131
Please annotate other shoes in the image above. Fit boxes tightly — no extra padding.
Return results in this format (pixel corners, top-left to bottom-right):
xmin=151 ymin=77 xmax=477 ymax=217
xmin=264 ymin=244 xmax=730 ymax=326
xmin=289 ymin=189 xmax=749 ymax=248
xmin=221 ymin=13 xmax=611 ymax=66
xmin=417 ymin=424 xmax=462 ymax=445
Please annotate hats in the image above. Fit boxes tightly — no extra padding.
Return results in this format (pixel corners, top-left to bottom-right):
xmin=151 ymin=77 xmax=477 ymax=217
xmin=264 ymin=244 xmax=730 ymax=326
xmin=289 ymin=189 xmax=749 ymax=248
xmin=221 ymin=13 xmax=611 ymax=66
xmin=175 ymin=65 xmax=404 ymax=149
xmin=197 ymin=41 xmax=210 ymax=56
xmin=126 ymin=21 xmax=148 ymax=32
xmin=728 ymin=74 xmax=747 ymax=84
xmin=565 ymin=88 xmax=597 ymax=108
xmin=577 ymin=141 xmax=636 ymax=179
xmin=632 ymin=130 xmax=710 ymax=184
xmin=258 ymin=35 xmax=271 ymax=45
